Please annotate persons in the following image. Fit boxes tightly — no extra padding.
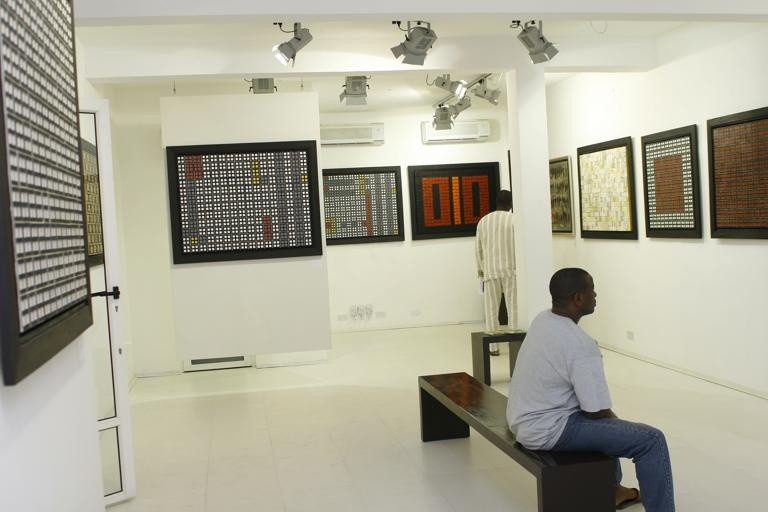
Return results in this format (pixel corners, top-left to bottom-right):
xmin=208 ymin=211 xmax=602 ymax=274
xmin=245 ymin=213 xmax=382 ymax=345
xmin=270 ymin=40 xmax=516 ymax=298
xmin=474 ymin=189 xmax=515 ymax=356
xmin=506 ymin=268 xmax=676 ymax=511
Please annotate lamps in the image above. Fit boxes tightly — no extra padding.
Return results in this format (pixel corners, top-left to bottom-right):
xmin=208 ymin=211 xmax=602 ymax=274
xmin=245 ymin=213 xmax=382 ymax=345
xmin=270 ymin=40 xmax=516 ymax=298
xmin=390 ymin=23 xmax=437 ymax=66
xmin=510 ymin=18 xmax=558 ymax=65
xmin=339 ymin=74 xmax=369 ymax=106
xmin=425 ymin=72 xmax=502 ymax=132
xmin=272 ymin=21 xmax=314 ymax=67
xmin=249 ymin=75 xmax=276 ymax=95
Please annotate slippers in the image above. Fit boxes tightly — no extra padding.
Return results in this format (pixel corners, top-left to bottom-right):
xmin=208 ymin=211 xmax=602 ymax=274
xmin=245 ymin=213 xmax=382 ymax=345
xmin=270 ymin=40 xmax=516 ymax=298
xmin=490 ymin=351 xmax=500 ymax=356
xmin=616 ymin=487 xmax=643 ymax=510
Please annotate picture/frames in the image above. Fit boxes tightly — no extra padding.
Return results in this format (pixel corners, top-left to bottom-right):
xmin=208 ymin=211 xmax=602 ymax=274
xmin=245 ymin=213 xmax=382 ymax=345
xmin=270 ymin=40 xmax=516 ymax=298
xmin=407 ymin=161 xmax=500 ymax=241
xmin=576 ymin=135 xmax=638 ymax=240
xmin=0 ymin=0 xmax=94 ymax=387
xmin=319 ymin=165 xmax=405 ymax=247
xmin=79 ymin=136 xmax=106 ymax=268
xmin=548 ymin=154 xmax=575 ymax=238
xmin=166 ymin=139 xmax=323 ymax=265
xmin=707 ymin=106 xmax=768 ymax=239
xmin=640 ymin=124 xmax=702 ymax=239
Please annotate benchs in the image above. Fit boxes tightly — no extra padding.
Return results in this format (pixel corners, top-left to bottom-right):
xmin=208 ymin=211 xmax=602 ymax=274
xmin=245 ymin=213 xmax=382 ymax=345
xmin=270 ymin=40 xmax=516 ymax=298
xmin=417 ymin=371 xmax=618 ymax=512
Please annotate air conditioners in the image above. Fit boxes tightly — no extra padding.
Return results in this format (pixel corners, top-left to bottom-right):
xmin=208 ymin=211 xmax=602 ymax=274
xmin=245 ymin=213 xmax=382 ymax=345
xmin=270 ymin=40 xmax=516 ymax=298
xmin=420 ymin=119 xmax=491 ymax=145
xmin=320 ymin=122 xmax=386 ymax=146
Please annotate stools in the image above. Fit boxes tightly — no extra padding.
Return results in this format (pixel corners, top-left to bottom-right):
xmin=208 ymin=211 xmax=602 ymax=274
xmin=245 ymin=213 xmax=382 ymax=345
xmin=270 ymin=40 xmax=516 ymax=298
xmin=470 ymin=328 xmax=527 ymax=386
